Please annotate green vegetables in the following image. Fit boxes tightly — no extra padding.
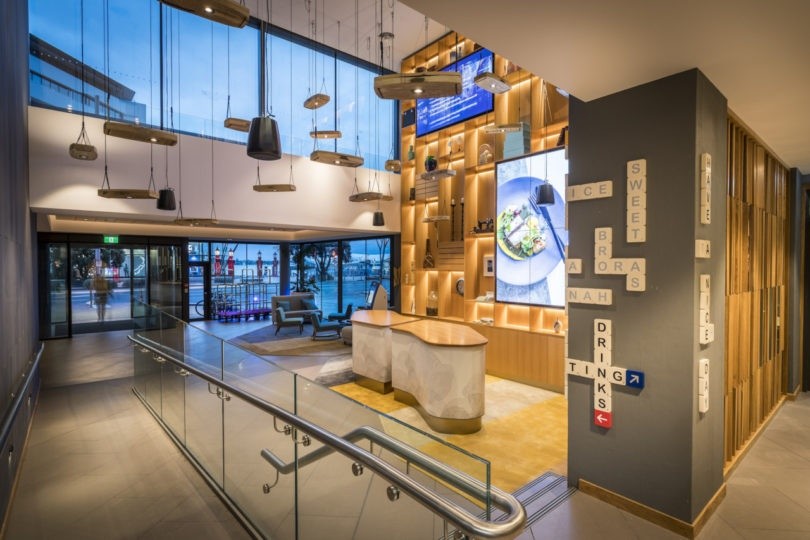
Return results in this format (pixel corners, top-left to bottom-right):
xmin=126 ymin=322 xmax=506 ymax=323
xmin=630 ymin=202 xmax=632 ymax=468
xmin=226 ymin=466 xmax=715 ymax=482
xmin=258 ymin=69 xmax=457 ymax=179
xmin=501 ymin=211 xmax=542 ymax=256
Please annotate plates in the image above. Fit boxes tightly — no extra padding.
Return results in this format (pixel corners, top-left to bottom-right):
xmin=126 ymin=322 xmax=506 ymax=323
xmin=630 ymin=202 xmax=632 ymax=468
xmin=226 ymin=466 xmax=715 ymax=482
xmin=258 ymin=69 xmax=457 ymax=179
xmin=497 ymin=176 xmax=566 ymax=286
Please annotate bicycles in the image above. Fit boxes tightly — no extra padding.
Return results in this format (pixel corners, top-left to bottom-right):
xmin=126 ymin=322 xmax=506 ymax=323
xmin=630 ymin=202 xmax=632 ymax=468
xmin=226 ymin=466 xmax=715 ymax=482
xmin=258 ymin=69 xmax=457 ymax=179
xmin=195 ymin=291 xmax=231 ymax=316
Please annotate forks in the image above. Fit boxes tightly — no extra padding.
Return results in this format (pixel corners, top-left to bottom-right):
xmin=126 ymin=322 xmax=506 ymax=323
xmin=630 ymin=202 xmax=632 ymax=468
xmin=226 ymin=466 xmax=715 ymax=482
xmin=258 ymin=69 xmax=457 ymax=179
xmin=528 ymin=192 xmax=565 ymax=251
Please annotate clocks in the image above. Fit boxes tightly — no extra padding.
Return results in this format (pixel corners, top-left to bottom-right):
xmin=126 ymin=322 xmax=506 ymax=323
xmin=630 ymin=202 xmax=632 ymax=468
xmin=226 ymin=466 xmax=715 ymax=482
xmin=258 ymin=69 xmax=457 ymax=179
xmin=456 ymin=278 xmax=464 ymax=296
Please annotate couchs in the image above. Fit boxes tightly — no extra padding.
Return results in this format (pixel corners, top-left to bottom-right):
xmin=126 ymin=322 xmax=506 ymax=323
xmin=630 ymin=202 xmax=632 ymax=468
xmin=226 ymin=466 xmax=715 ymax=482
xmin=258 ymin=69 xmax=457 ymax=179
xmin=271 ymin=294 xmax=322 ymax=326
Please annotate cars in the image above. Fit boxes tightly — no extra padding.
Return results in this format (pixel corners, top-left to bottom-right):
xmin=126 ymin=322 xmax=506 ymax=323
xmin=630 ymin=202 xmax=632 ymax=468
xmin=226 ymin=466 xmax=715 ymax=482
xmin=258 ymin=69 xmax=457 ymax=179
xmin=343 ymin=258 xmax=391 ymax=277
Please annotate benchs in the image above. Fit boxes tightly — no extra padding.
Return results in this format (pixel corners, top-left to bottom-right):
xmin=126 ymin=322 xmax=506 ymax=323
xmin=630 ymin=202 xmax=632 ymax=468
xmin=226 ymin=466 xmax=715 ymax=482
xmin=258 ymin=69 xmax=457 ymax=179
xmin=275 ymin=307 xmax=304 ymax=336
xmin=328 ymin=303 xmax=353 ymax=322
xmin=311 ymin=311 xmax=341 ymax=340
xmin=342 ymin=326 xmax=353 ymax=347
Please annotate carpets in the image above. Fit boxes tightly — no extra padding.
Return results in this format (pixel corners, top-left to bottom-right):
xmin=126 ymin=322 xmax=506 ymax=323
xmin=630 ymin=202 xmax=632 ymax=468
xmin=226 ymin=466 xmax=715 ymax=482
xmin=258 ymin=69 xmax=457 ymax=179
xmin=227 ymin=318 xmax=352 ymax=356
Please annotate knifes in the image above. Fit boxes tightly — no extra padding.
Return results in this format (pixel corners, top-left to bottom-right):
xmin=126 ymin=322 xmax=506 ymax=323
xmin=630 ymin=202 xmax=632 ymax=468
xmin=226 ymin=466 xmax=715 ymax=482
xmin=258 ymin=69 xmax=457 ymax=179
xmin=536 ymin=186 xmax=566 ymax=262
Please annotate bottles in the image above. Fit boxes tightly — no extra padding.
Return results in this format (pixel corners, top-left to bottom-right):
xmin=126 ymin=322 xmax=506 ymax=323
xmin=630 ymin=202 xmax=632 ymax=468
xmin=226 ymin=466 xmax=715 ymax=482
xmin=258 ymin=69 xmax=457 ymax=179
xmin=408 ymin=146 xmax=414 ymax=161
xmin=423 ymin=238 xmax=434 ymax=268
xmin=426 ymin=291 xmax=438 ymax=316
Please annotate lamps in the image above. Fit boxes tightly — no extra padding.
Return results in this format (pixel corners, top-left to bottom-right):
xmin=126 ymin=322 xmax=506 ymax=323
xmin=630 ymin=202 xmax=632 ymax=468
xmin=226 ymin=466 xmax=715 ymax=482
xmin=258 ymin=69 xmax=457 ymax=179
xmin=69 ymin=0 xmax=529 ymax=228
xmin=257 ymin=249 xmax=262 ymax=258
xmin=215 ymin=247 xmax=220 ymax=256
xmin=274 ymin=252 xmax=277 ymax=259
xmin=229 ymin=248 xmax=233 ymax=256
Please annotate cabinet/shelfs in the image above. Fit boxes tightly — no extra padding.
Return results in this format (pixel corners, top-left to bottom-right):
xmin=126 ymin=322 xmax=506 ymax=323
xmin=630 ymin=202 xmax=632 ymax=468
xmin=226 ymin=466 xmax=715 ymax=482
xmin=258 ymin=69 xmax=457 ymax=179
xmin=399 ymin=30 xmax=569 ymax=395
xmin=217 ymin=282 xmax=278 ymax=323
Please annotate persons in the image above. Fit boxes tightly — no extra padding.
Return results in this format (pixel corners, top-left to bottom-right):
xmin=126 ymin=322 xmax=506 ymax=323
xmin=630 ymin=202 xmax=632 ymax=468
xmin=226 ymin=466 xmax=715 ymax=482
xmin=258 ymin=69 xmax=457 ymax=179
xmin=90 ymin=266 xmax=114 ymax=322
xmin=123 ymin=265 xmax=129 ymax=277
